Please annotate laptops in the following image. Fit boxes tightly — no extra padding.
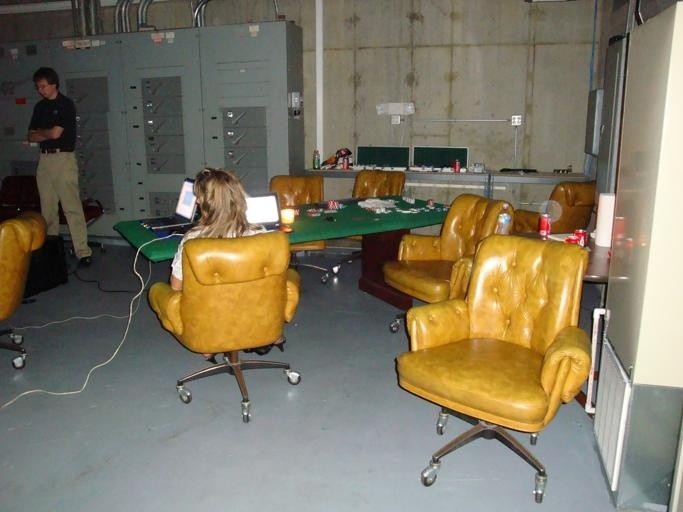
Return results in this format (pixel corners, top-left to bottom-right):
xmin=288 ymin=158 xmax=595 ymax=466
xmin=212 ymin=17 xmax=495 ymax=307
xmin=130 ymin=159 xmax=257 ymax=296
xmin=241 ymin=191 xmax=282 ymax=230
xmin=139 ymin=178 xmax=199 ymax=230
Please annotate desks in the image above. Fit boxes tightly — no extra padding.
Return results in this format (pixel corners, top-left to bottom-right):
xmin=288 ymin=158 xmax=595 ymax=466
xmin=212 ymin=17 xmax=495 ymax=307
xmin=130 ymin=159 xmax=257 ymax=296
xmin=522 ymin=233 xmax=611 ymax=417
xmin=302 ymin=164 xmax=591 ymax=236
xmin=112 ymin=196 xmax=449 ymax=311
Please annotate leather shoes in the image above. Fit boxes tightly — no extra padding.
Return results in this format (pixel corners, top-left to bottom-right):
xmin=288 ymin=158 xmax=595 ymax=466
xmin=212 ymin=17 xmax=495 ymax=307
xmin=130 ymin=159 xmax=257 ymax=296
xmin=79 ymin=256 xmax=92 ymax=266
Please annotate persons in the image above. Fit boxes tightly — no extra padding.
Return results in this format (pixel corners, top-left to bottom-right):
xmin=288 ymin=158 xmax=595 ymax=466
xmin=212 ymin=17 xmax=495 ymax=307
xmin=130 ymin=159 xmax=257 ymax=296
xmin=170 ymin=168 xmax=272 ymax=292
xmin=27 ymin=67 xmax=93 ymax=267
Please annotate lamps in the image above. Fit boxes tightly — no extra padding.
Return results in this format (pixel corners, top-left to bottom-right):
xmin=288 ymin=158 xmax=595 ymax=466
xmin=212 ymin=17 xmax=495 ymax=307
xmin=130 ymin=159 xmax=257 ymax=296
xmin=278 ymin=209 xmax=295 ymax=233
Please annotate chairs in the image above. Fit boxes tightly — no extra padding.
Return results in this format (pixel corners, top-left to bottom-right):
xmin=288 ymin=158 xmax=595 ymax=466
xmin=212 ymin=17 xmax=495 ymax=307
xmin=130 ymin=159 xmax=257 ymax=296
xmin=514 ymin=182 xmax=596 ymax=238
xmin=0 ymin=175 xmax=37 ymax=216
xmin=148 ymin=231 xmax=302 ymax=422
xmin=268 ymin=175 xmax=329 ymax=284
xmin=381 ymin=193 xmax=513 ymax=333
xmin=0 ymin=212 xmax=47 ymax=369
xmin=394 ymin=234 xmax=590 ymax=502
xmin=33 ymin=183 xmax=106 ymax=257
xmin=333 ymin=170 xmax=406 ymax=274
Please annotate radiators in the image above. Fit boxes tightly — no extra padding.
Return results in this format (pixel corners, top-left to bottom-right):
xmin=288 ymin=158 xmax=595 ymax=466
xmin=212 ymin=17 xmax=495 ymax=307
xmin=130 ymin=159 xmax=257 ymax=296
xmin=592 ymin=338 xmax=631 ymax=491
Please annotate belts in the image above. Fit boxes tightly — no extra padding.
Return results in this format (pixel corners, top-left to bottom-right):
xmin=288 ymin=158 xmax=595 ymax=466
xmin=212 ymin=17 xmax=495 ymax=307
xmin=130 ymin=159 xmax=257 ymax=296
xmin=39 ymin=148 xmax=73 ymax=154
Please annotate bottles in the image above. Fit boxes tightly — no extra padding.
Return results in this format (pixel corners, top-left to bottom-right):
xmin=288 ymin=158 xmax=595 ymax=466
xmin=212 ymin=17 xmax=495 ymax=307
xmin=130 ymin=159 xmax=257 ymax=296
xmin=454 ymin=159 xmax=461 ymax=173
xmin=497 ymin=203 xmax=510 ymax=235
xmin=626 ymin=239 xmax=632 ymax=250
xmin=344 ymin=157 xmax=349 ymax=170
xmin=615 ymin=218 xmax=624 ymax=245
xmin=540 ymin=214 xmax=550 ymax=237
xmin=575 ymin=229 xmax=588 ymax=247
xmin=313 ymin=149 xmax=320 ymax=170
xmin=566 ymin=236 xmax=580 ymax=244
